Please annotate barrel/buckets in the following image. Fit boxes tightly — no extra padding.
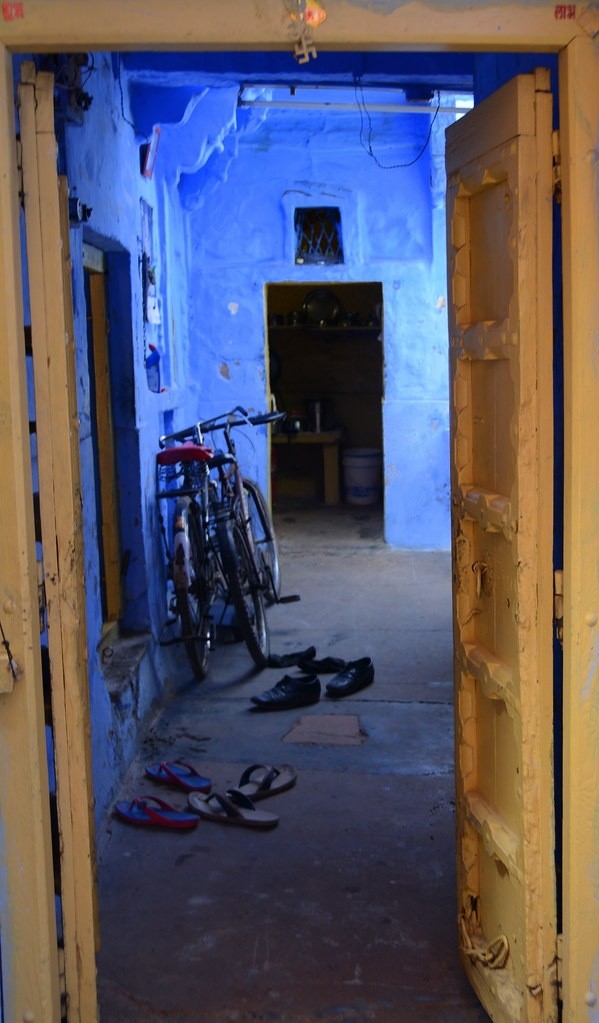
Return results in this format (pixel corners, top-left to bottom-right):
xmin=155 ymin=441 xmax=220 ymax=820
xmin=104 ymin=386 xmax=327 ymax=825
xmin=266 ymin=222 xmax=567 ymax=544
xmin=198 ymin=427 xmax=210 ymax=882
xmin=342 ymin=447 xmax=381 ymax=505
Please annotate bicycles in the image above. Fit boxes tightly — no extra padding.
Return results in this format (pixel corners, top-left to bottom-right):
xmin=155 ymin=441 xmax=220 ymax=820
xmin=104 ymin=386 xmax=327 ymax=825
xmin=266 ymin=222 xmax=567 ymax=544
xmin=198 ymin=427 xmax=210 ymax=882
xmin=155 ymin=405 xmax=252 ymax=683
xmin=172 ymin=407 xmax=302 ymax=667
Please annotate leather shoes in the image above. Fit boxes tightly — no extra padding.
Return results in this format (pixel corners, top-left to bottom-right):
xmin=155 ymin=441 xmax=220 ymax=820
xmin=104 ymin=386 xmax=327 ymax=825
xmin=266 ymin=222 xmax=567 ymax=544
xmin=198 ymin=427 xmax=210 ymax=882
xmin=326 ymin=657 xmax=374 ymax=694
xmin=250 ymin=675 xmax=321 ymax=708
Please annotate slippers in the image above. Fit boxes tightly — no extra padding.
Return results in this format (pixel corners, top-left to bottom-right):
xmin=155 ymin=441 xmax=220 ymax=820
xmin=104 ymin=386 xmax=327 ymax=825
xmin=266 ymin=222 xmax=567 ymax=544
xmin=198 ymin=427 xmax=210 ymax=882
xmin=114 ymin=795 xmax=200 ymax=827
xmin=146 ymin=760 xmax=212 ymax=793
xmin=225 ymin=763 xmax=298 ymax=801
xmin=189 ymin=789 xmax=279 ymax=826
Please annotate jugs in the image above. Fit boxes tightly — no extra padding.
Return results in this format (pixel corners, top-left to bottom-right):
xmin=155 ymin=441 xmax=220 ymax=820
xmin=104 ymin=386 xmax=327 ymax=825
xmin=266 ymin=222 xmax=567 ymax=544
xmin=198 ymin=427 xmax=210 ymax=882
xmin=303 ymin=397 xmax=336 ymax=432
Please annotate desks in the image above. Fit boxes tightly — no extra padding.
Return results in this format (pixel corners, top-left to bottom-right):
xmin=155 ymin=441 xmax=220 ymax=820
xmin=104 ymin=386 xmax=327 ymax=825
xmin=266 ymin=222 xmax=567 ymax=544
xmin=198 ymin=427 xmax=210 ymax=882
xmin=272 ymin=429 xmax=342 ymax=506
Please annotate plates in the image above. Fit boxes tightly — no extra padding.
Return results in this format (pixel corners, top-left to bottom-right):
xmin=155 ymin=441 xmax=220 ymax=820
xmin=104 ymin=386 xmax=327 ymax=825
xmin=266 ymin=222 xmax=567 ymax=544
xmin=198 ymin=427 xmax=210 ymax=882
xmin=302 ymin=289 xmax=339 ymax=323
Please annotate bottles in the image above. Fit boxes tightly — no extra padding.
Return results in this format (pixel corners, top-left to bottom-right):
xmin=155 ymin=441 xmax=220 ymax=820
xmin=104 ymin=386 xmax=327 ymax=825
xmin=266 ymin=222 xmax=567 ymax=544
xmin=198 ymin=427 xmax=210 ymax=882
xmin=289 ymin=410 xmax=302 ymax=433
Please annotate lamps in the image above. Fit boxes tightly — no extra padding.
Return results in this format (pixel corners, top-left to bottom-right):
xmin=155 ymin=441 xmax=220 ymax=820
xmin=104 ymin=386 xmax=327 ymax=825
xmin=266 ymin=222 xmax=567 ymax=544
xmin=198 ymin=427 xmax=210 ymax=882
xmin=235 ymin=83 xmax=472 ymax=116
